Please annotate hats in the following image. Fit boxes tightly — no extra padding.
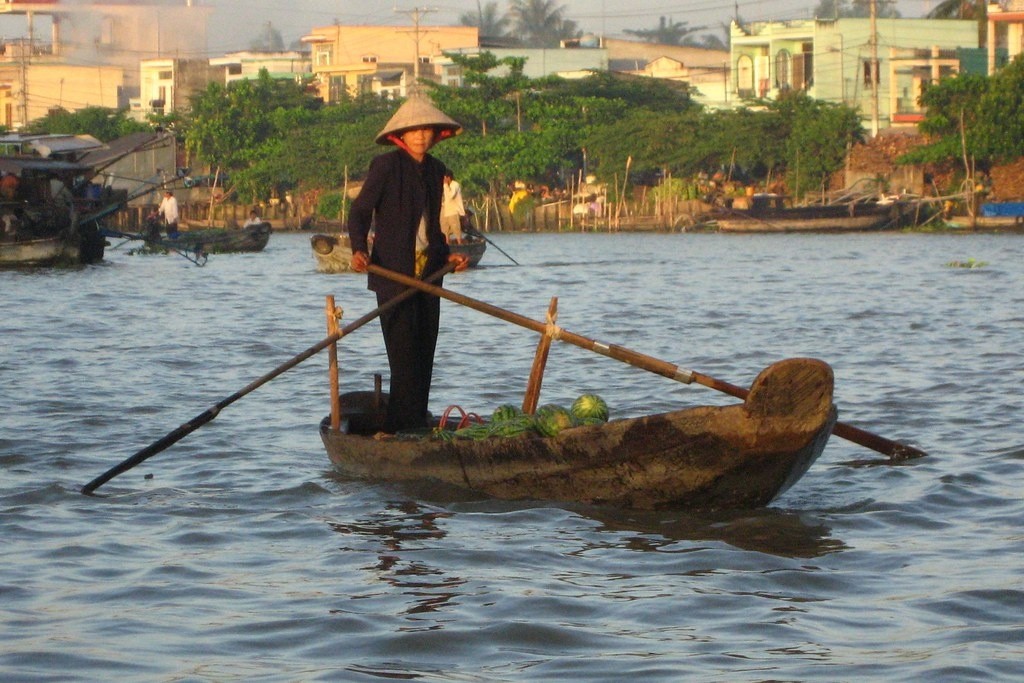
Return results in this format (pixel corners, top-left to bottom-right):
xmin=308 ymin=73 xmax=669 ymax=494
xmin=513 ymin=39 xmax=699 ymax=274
xmin=373 ymin=97 xmax=462 ymax=146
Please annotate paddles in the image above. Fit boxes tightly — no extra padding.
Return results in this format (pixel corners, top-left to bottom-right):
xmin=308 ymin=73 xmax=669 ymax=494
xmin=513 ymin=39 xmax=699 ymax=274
xmin=363 ymin=262 xmax=929 ymax=460
xmin=79 ymin=260 xmax=460 ymax=495
xmin=462 ymin=224 xmax=519 ymax=265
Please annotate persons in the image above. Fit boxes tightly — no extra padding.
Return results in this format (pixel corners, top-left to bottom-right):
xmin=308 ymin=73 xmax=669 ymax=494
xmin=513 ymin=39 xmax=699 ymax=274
xmin=243 ymin=210 xmax=261 ymax=229
xmin=348 ymin=96 xmax=471 ymax=433
xmin=439 ymin=168 xmax=466 ymax=244
xmin=152 ymin=191 xmax=179 ymax=240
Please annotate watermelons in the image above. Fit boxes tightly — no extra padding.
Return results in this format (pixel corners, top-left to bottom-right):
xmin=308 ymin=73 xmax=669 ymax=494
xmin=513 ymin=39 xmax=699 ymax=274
xmin=430 ymin=394 xmax=609 ymax=441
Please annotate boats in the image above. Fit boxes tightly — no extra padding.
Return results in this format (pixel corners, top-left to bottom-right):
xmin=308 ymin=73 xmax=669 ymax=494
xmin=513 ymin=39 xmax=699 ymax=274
xmin=713 ymin=192 xmax=893 ymax=231
xmin=310 ymin=232 xmax=488 ymax=272
xmin=320 ymin=355 xmax=839 ymax=511
xmin=0 ymin=154 xmax=110 ymax=268
xmin=143 ymin=221 xmax=273 ymax=254
